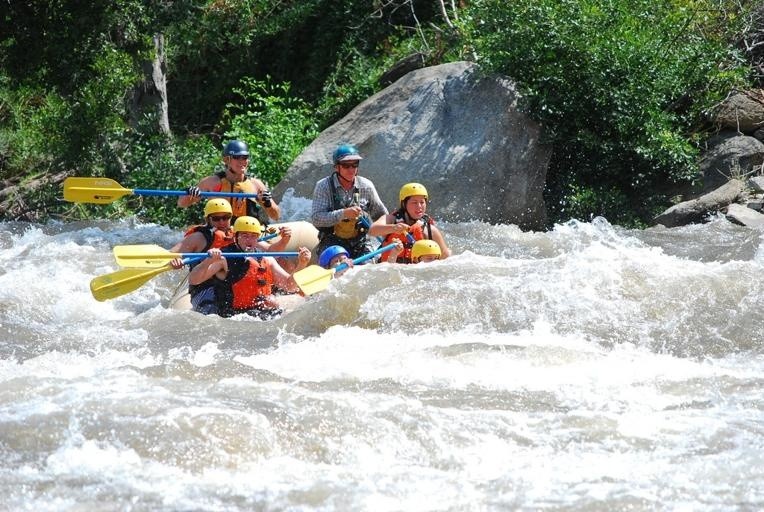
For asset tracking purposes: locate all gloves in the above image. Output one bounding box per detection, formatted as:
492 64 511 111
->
187 185 202 198
258 190 273 207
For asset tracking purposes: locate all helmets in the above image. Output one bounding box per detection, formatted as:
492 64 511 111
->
400 182 429 203
319 244 350 268
233 215 261 237
205 197 233 219
221 140 250 156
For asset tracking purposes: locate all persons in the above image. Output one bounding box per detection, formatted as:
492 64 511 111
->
168 197 292 315
317 238 405 278
187 215 312 322
178 139 281 225
177 241 181 245
368 182 450 264
311 144 390 264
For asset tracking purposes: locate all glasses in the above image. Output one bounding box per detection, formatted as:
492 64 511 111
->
209 213 230 222
339 162 359 168
232 154 249 160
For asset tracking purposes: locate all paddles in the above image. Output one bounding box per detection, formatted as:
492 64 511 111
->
292 242 397 297
62 176 258 204
112 244 300 269
89 231 281 302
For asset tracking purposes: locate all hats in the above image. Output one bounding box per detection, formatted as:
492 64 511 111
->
333 144 363 163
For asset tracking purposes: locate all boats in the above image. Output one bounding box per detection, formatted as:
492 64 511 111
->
168 221 321 310
